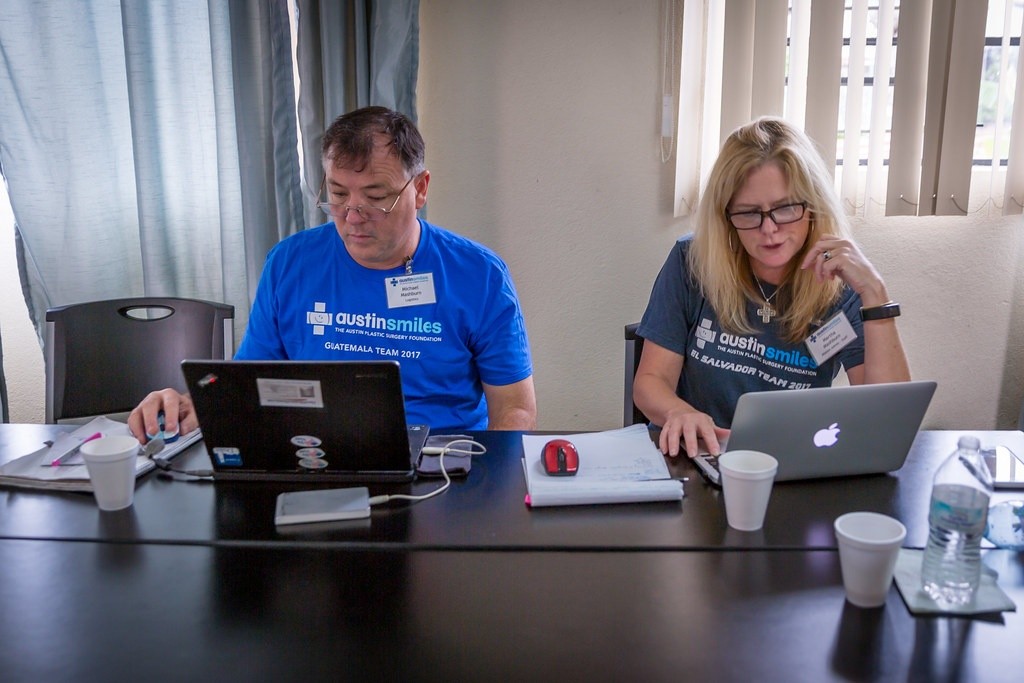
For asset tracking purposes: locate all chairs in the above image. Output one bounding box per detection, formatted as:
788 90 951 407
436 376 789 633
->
624 322 651 427
43 296 235 425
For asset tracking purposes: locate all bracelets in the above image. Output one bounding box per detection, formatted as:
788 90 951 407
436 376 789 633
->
859 300 901 322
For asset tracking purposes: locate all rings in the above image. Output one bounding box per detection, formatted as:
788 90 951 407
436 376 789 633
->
823 249 832 261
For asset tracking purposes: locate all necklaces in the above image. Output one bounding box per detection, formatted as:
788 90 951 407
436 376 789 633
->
751 266 789 324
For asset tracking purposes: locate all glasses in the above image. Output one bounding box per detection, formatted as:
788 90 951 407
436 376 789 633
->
725 201 808 230
315 173 414 221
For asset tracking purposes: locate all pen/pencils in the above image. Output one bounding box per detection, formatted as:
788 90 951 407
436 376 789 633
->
48 432 102 466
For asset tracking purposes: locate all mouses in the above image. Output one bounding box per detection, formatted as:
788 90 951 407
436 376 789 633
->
146 409 180 444
540 439 579 476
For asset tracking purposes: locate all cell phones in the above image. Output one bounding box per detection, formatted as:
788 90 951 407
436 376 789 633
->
959 445 1024 489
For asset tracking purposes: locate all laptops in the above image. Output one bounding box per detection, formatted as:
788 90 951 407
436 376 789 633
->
681 380 938 487
180 359 428 483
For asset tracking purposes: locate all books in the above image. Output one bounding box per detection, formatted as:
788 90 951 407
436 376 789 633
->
521 423 684 506
0 415 203 491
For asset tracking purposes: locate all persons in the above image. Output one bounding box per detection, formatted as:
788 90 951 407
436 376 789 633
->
633 118 912 457
129 106 537 443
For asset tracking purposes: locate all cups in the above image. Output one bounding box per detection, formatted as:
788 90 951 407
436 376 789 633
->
718 450 778 531
834 512 907 608
80 436 140 511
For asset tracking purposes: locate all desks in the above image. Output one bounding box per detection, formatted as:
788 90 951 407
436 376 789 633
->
0 423 1024 683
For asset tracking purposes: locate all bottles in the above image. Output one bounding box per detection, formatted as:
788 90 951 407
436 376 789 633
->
922 435 994 605
982 500 1024 551
